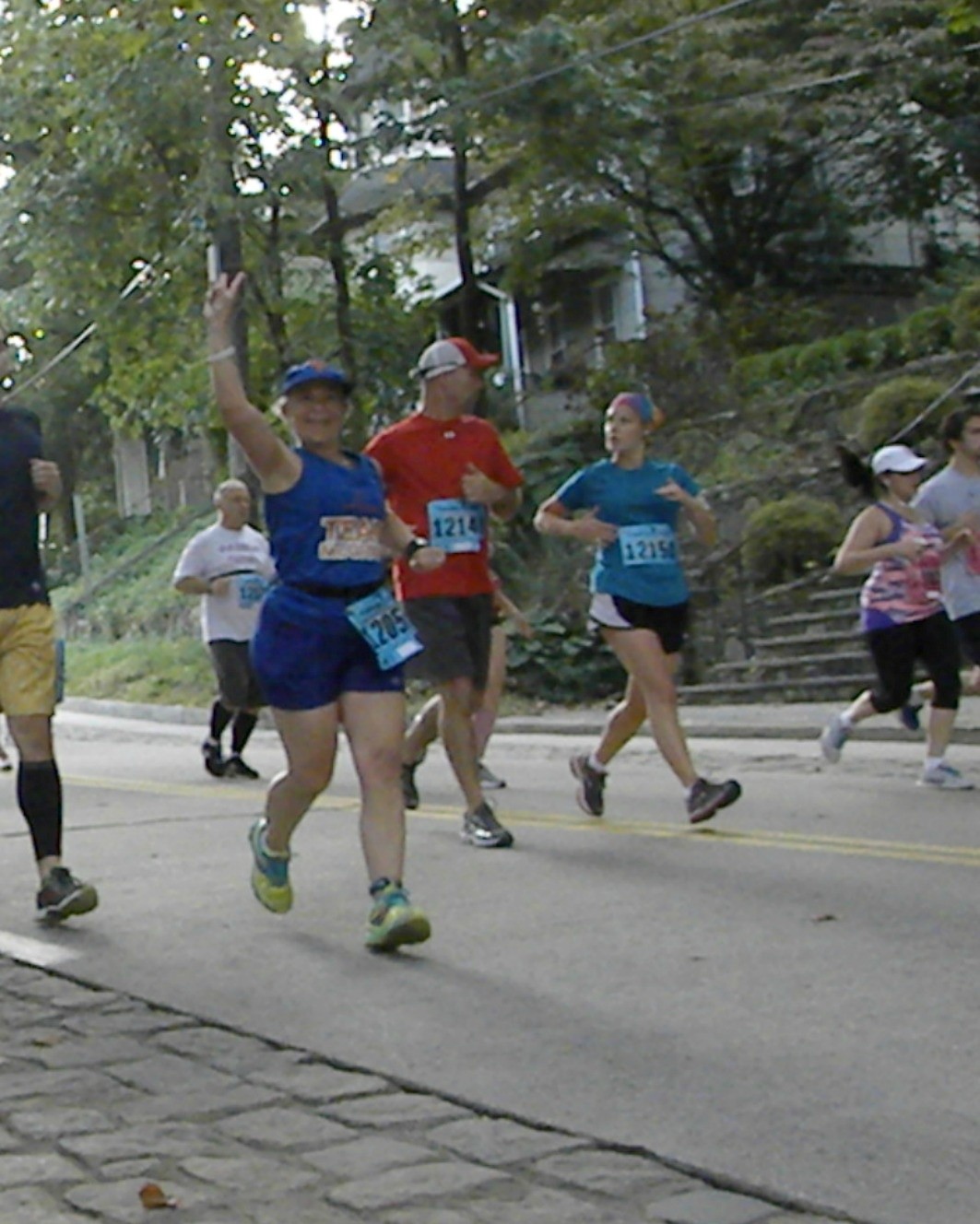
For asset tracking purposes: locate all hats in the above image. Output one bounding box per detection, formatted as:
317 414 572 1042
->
408 336 502 380
871 443 930 477
282 359 351 394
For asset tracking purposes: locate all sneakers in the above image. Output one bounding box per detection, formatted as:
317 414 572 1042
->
569 750 608 816
247 816 295 914
683 778 742 824
917 761 973 791
201 741 225 776
363 878 431 949
402 763 419 809
459 803 514 847
33 865 99 923
815 715 852 765
224 757 259 780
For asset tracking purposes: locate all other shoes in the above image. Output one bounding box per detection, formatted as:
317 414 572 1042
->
1 749 14 773
476 763 506 790
414 747 427 766
902 703 924 731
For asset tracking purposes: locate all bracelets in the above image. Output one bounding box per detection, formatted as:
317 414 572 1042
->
208 344 236 363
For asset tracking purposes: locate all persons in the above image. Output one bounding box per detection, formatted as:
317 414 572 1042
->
170 479 282 779
359 337 527 847
204 271 431 951
533 391 741 823
819 407 980 790
0 406 100 923
